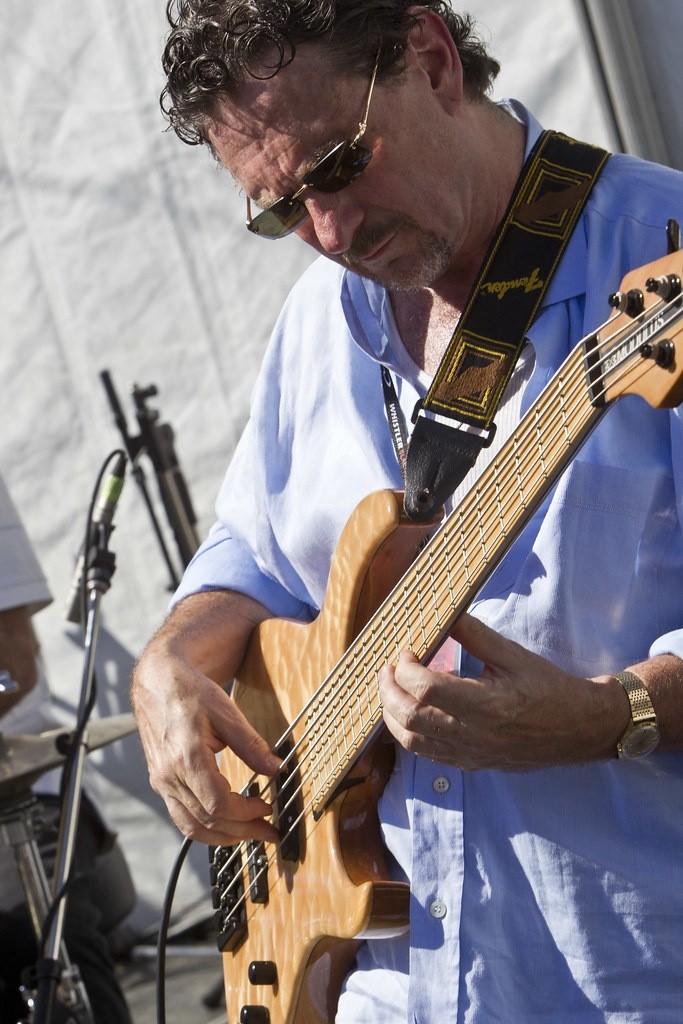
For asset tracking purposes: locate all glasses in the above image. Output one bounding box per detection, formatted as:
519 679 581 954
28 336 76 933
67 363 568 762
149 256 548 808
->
245 34 382 240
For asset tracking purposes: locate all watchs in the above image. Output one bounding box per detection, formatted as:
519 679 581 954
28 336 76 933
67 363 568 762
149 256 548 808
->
610 671 661 762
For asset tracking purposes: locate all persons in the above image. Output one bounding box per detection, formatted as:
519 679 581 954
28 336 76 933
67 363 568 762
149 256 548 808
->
1 478 98 1024
128 0 683 1024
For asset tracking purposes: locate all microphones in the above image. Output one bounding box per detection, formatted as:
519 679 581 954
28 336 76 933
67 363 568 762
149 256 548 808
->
65 454 131 624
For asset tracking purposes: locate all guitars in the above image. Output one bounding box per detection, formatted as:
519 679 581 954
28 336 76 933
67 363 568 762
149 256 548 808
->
208 221 683 1024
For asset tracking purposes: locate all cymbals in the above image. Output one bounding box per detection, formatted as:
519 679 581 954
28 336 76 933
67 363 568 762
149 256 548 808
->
0 709 140 809
